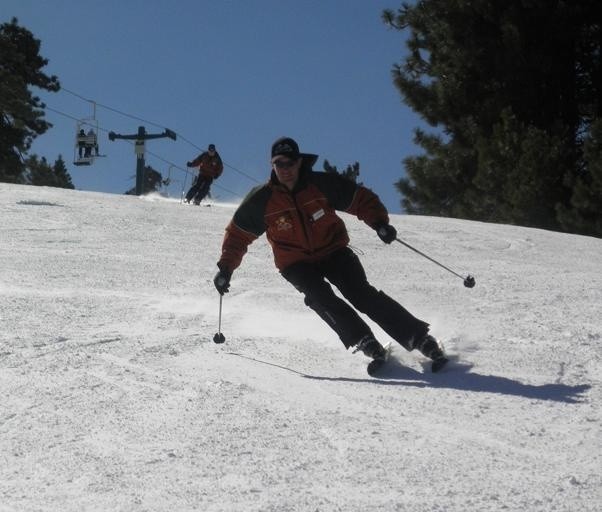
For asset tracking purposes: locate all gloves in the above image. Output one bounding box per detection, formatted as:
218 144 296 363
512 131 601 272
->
214 271 232 296
187 162 192 167
372 220 397 244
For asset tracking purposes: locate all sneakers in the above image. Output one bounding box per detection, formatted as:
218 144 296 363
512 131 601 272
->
421 337 451 373
359 336 388 376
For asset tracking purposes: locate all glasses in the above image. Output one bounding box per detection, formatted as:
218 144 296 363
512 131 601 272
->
275 160 295 168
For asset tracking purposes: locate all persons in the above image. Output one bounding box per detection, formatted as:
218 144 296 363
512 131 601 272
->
184 145 223 205
214 139 443 363
78 129 97 155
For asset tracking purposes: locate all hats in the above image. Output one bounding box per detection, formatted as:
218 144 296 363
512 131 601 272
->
272 136 300 161
209 144 216 152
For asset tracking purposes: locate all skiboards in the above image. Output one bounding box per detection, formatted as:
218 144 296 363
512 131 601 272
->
367 339 449 376
